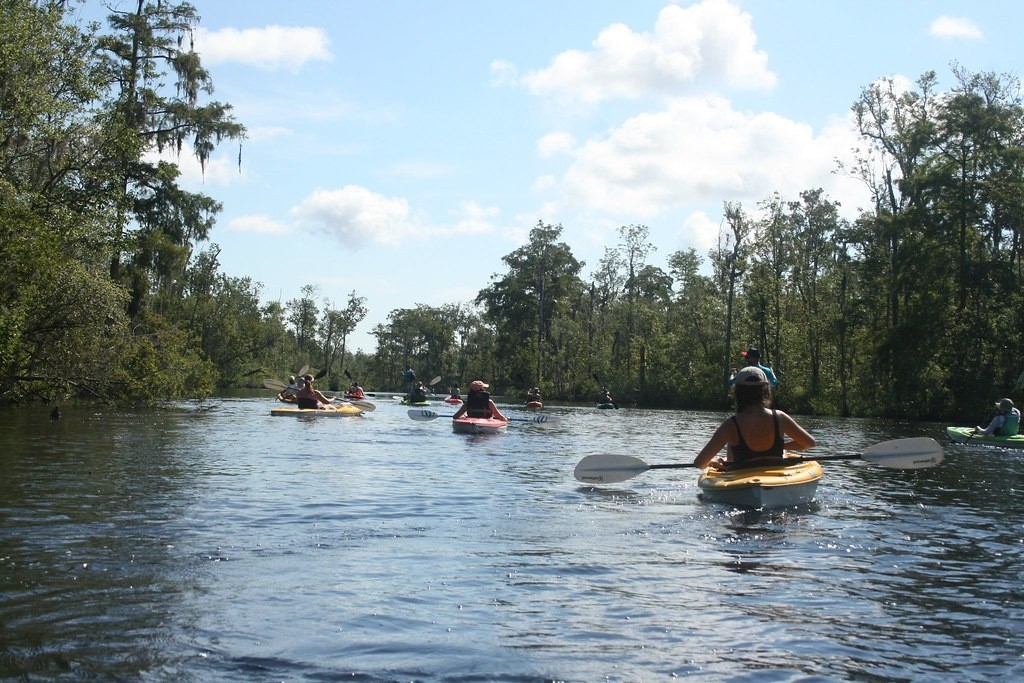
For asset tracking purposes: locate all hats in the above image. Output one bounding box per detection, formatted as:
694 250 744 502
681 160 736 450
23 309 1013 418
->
735 366 768 386
742 348 760 358
289 376 295 380
471 381 489 392
353 382 359 386
415 381 423 389
996 398 1014 411
303 375 314 382
532 388 540 393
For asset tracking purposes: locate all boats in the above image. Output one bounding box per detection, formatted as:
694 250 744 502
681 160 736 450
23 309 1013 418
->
271 402 363 416
445 395 463 404
947 426 1024 449
451 415 508 434
343 393 366 400
698 449 823 510
526 401 543 410
400 400 429 406
597 403 614 410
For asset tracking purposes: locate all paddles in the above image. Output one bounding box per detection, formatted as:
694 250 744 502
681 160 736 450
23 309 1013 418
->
593 373 619 409
344 369 352 380
399 375 442 405
314 370 328 379
963 406 998 445
365 393 375 397
573 432 947 486
407 409 561 429
275 364 310 401
263 378 377 412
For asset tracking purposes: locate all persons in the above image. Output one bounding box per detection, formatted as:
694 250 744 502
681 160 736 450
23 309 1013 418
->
453 381 508 421
728 348 778 389
599 387 612 402
693 367 817 469
297 374 337 409
401 365 461 401
280 375 365 399
976 398 1021 437
526 387 543 402
